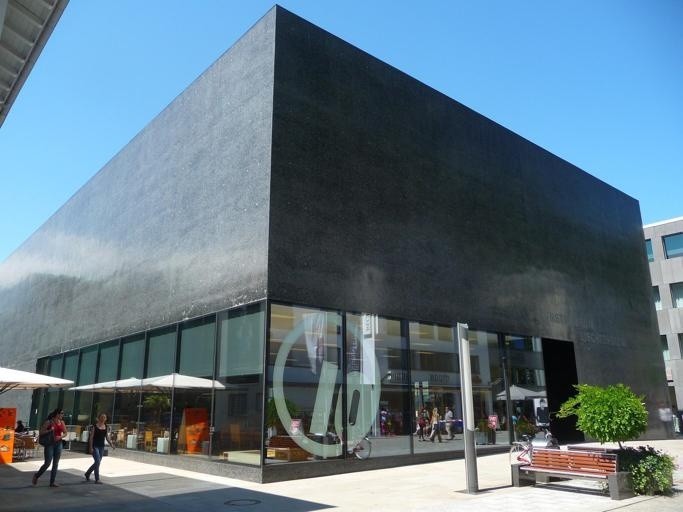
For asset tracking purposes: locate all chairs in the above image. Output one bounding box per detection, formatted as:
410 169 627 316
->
60 431 89 453
13 433 39 460
228 423 247 450
143 429 157 452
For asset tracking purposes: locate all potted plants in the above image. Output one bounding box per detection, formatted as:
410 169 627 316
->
514 417 538 441
629 454 676 496
474 419 496 445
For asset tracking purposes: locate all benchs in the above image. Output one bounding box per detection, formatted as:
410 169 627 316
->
510 444 635 499
265 434 308 461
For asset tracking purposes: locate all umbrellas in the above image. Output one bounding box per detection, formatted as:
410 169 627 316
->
493 384 546 401
139 372 226 392
0 367 75 394
67 376 158 393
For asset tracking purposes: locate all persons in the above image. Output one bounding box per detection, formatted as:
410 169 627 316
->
15 420 24 431
32 407 68 487
84 411 116 485
658 403 676 441
676 411 683 434
518 413 526 432
536 399 550 426
512 415 517 432
380 407 455 444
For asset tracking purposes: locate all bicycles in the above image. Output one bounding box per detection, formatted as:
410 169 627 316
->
509 426 561 465
349 422 372 461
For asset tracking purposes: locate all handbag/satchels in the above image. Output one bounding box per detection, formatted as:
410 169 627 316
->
38 420 55 447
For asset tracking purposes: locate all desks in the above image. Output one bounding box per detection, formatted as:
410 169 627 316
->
156 437 169 454
126 434 137 449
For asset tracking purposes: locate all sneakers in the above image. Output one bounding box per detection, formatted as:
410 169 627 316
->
94 479 102 485
84 472 91 481
31 473 39 487
49 482 59 487
